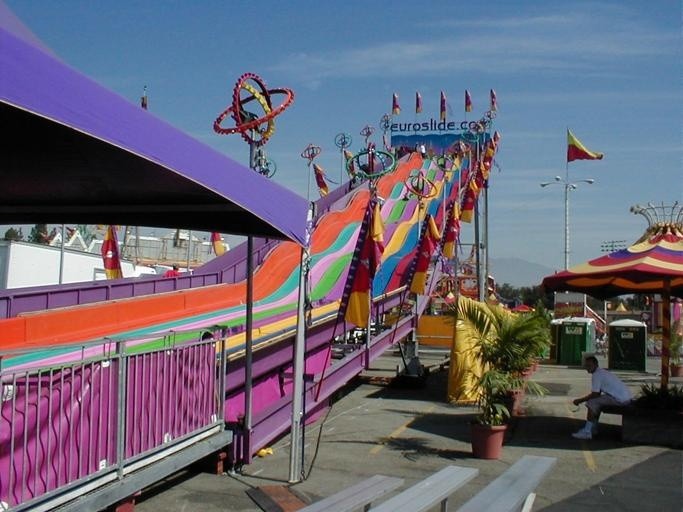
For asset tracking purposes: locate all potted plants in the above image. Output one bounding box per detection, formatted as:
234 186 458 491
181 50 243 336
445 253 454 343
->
669 318 683 377
429 285 555 460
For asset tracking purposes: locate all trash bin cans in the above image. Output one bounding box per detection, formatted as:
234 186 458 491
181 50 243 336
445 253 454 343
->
609 318 647 372
551 319 561 360
560 317 596 366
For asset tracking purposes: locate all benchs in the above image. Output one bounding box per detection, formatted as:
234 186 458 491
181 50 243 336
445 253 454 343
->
295 455 557 512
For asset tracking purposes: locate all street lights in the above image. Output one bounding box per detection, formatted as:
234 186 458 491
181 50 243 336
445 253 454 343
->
541 175 595 271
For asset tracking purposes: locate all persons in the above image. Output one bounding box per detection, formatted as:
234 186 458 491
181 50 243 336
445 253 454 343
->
568 355 632 439
162 263 181 277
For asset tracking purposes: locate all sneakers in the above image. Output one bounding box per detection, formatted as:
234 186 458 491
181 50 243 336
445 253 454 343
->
572 430 591 440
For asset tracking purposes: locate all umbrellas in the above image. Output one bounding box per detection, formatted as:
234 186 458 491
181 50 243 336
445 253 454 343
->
539 220 682 391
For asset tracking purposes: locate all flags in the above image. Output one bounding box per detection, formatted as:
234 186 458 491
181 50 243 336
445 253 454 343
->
381 133 392 154
344 150 356 183
490 90 498 114
567 128 603 165
310 163 328 198
440 90 445 122
335 198 386 330
401 212 441 298
391 94 400 116
458 130 499 225
464 89 472 112
437 198 461 260
99 225 124 280
211 232 225 257
414 90 422 114
